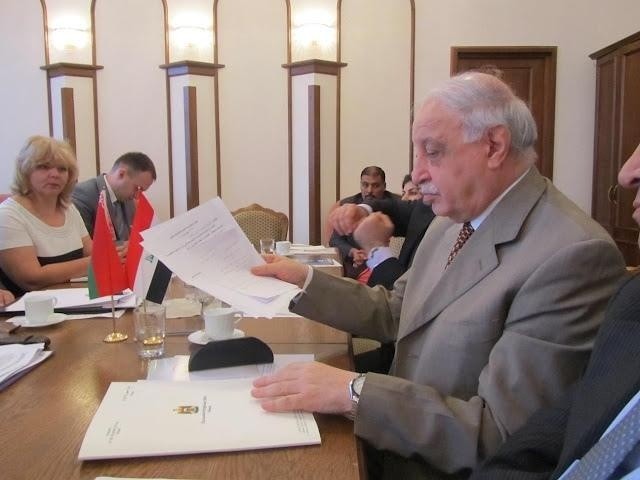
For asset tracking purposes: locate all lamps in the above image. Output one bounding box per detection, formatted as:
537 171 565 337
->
283 0 342 64
38 0 97 68
162 1 220 66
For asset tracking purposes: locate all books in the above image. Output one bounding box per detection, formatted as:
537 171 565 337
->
78 381 321 460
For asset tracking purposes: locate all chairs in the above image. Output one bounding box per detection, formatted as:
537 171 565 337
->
229 202 289 253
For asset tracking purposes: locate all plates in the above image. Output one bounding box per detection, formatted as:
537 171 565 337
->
14 312 68 329
273 252 296 258
188 326 246 347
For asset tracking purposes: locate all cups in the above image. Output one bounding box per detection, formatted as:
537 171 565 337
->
203 308 247 342
259 238 274 257
277 241 295 255
196 289 223 320
21 292 57 325
132 303 169 360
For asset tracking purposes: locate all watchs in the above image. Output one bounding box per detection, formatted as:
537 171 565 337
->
346 372 367 422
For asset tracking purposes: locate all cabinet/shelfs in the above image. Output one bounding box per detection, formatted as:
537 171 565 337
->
586 31 639 272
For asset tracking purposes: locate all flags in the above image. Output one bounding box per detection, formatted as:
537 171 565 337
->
87 191 129 299
124 192 174 304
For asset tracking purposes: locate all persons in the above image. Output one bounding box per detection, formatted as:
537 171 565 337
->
0 136 129 292
0 281 15 309
467 144 640 480
74 152 156 240
324 165 436 374
250 68 627 480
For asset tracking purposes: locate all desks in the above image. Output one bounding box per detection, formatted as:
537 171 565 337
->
0 241 355 346
0 337 368 480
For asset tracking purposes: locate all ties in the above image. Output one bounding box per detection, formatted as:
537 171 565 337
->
562 396 637 473
448 222 472 267
